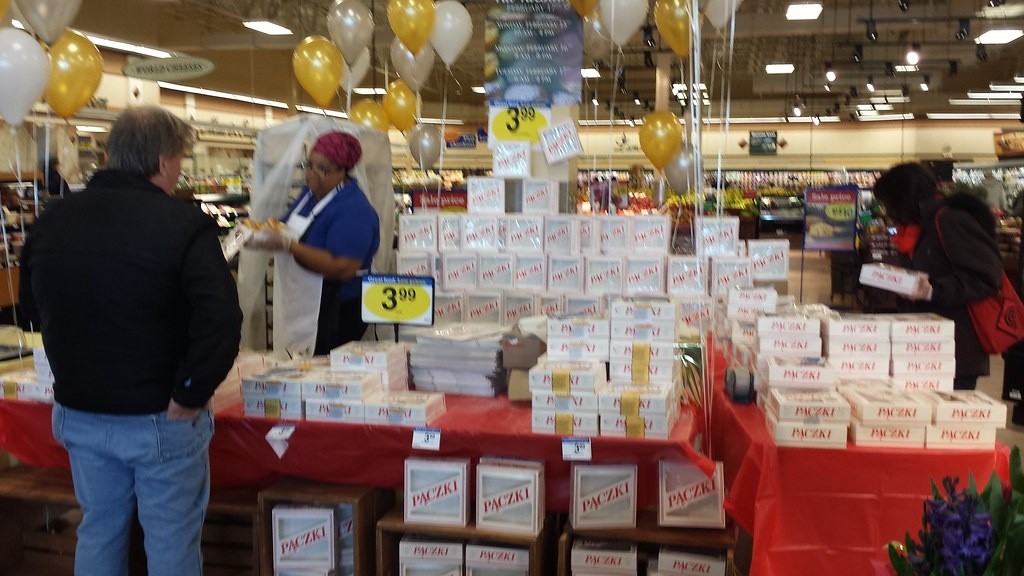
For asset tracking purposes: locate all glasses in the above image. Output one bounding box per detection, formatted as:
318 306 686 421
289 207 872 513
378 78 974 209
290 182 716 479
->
301 160 339 175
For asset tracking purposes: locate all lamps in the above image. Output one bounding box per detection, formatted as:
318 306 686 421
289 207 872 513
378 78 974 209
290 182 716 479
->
852 44 865 63
906 42 922 65
640 24 659 47
11 18 172 59
898 0 911 12
765 64 796 74
866 75 875 93
955 18 970 40
785 3 823 21
591 91 600 107
919 72 930 92
867 19 879 41
811 113 821 126
976 43 990 63
824 62 837 82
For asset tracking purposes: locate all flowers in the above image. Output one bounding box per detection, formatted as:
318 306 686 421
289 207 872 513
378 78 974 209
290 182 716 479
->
888 445 1024 576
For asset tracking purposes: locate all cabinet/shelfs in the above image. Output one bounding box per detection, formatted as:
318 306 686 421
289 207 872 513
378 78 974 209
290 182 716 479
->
375 509 541 576
0 465 258 576
0 107 1024 271
256 484 395 576
557 515 739 576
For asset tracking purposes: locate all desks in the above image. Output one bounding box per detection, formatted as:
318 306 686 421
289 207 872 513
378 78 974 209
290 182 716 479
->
0 381 717 491
702 331 1011 576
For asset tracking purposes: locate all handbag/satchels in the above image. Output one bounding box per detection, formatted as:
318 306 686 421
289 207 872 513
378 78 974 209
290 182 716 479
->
936 206 1024 354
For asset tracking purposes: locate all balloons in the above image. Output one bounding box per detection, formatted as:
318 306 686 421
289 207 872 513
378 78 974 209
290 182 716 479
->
350 97 388 133
704 0 742 29
331 41 371 94
389 36 435 92
664 141 704 196
386 0 435 55
654 0 694 59
292 35 343 108
571 0 649 61
0 0 103 127
428 0 472 66
409 124 441 170
382 80 423 132
327 0 374 66
639 110 682 175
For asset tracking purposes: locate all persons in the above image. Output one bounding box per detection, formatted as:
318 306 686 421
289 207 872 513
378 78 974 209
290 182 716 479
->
235 130 381 358
17 106 244 576
42 156 72 200
871 161 1002 389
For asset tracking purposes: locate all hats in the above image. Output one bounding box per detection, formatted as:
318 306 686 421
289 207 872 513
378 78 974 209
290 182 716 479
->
313 131 362 171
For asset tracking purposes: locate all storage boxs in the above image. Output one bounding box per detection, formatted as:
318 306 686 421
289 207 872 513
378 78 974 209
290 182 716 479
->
221 178 1006 451
